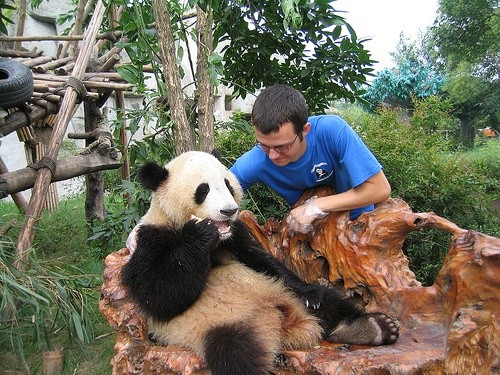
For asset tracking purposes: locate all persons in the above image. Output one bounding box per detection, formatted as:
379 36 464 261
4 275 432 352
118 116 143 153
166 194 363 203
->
126 84 392 256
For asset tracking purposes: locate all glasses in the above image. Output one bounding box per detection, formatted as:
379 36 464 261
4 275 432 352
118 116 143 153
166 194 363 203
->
254 128 304 154
